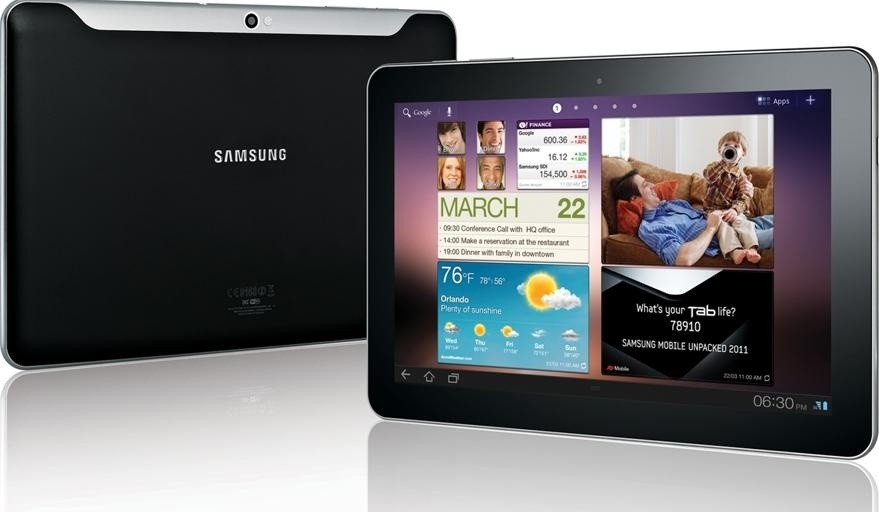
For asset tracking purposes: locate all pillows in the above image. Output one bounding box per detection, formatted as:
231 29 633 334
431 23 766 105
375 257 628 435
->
602 154 773 234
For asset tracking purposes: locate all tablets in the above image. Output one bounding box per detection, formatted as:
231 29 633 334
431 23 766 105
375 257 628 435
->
365 45 879 459
0 0 456 371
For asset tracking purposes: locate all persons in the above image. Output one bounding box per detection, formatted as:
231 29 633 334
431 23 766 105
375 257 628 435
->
610 168 774 267
477 121 505 155
438 158 466 190
477 157 505 191
702 132 762 264
437 122 466 155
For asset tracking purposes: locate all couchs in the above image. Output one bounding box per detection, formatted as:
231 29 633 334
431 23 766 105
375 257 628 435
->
601 156 774 270
